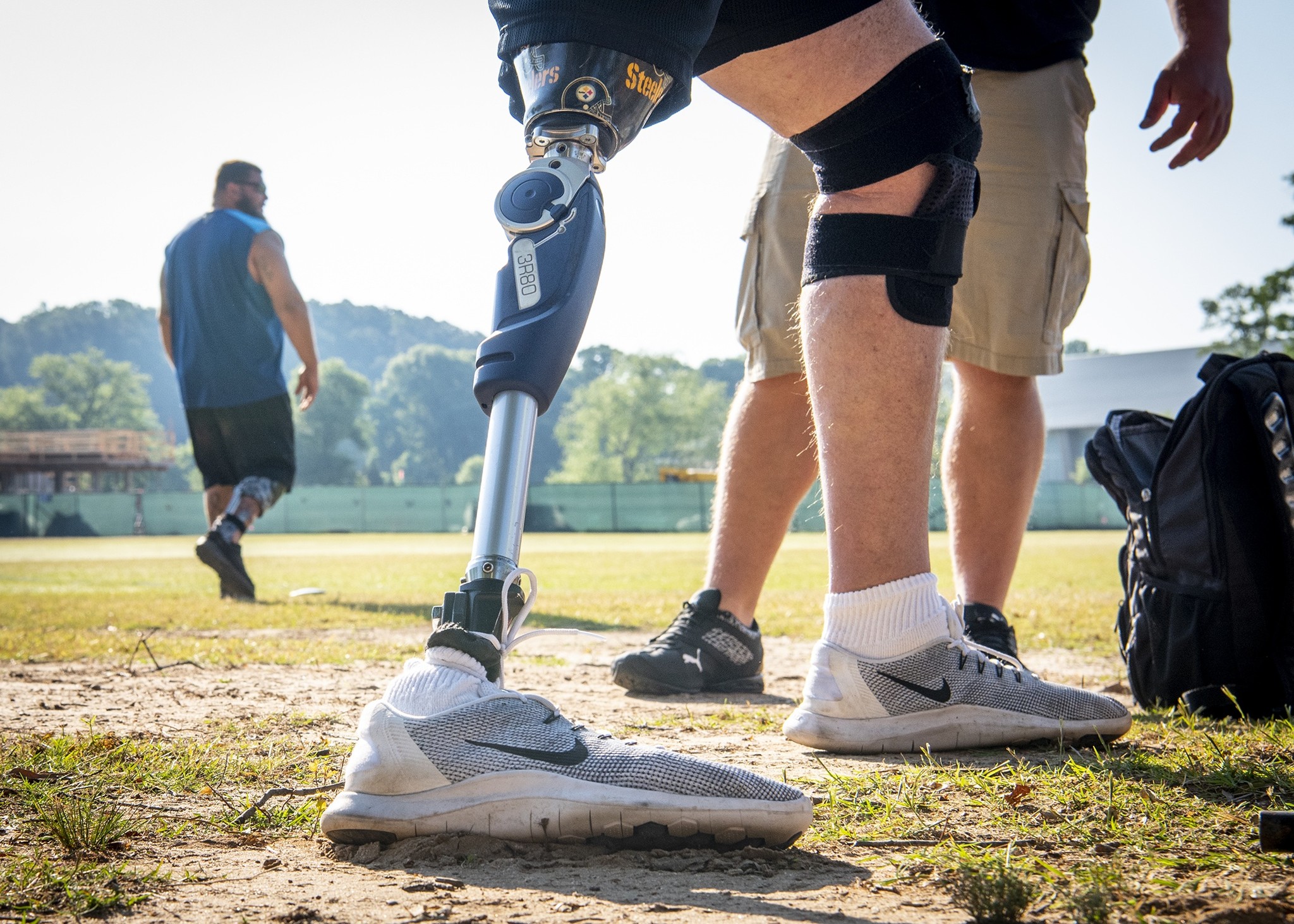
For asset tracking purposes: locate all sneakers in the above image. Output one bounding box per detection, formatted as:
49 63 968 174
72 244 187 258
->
781 595 1132 752
611 586 766 694
962 603 1018 664
321 567 813 857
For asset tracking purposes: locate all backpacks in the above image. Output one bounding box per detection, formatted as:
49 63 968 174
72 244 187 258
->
1085 346 1294 729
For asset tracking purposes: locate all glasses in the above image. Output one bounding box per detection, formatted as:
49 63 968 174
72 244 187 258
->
234 180 266 195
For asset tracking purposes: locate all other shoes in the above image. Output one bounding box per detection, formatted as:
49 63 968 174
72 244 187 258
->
194 529 256 600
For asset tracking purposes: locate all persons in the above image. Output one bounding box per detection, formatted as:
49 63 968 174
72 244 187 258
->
320 0 1132 847
159 159 323 603
607 1 1235 701
